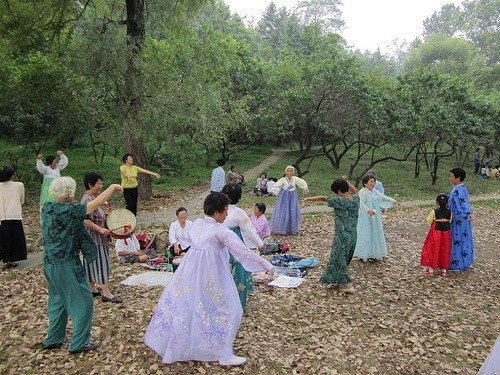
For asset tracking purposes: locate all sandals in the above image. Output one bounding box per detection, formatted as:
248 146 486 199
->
422 270 435 277
440 270 448 277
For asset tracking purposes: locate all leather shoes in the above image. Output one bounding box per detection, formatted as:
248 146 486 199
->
69 344 98 354
6 262 19 268
92 289 103 297
43 343 64 349
101 295 125 303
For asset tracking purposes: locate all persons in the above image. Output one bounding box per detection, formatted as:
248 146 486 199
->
222 184 270 317
270 165 309 236
210 159 227 192
40 176 124 353
81 171 123 303
447 167 475 271
227 165 246 186
475 148 482 173
115 234 157 264
254 173 279 197
168 207 192 273
144 192 276 366
0 166 27 269
36 150 68 225
481 161 500 180
120 153 161 216
420 193 452 277
365 171 385 213
300 175 361 293
354 175 400 263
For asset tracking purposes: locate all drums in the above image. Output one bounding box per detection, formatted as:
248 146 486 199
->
105 208 136 238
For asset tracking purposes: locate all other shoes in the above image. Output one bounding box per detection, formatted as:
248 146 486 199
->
368 257 377 263
219 355 248 366
339 287 357 294
